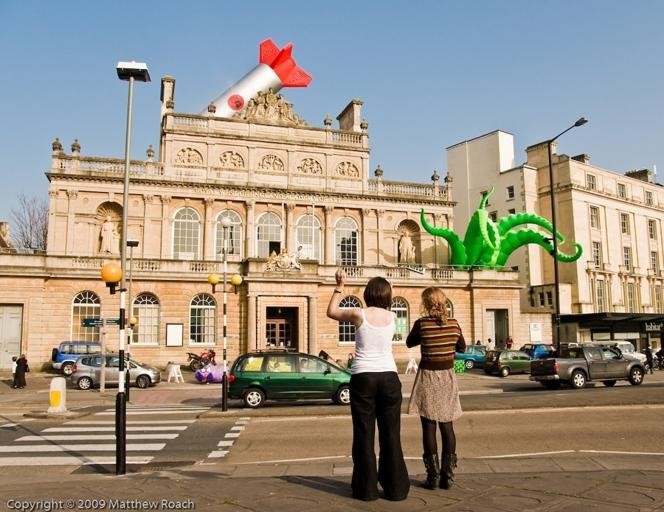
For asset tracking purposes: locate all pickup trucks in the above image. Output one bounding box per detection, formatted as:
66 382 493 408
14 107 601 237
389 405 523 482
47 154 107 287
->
528 344 645 394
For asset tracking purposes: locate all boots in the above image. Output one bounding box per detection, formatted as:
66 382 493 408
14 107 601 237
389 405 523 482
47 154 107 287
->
439 454 457 489
421 454 440 490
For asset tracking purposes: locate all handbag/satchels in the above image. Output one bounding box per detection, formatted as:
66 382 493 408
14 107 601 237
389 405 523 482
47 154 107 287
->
25 365 29 372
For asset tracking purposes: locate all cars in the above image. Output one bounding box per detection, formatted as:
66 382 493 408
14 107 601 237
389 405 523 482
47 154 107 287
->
452 343 488 371
519 341 552 360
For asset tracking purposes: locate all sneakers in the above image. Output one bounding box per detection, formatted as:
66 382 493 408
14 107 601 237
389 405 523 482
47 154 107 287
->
13 385 24 388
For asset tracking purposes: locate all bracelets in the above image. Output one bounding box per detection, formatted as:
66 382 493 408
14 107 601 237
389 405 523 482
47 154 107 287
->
333 289 341 293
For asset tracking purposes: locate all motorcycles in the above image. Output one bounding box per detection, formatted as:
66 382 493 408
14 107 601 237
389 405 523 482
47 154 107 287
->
185 348 216 371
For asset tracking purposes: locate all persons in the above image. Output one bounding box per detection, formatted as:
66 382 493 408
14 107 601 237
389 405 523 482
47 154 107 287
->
405 285 467 490
286 338 297 353
505 336 514 349
231 87 307 127
398 230 416 264
99 215 117 253
11 356 18 386
273 362 281 372
12 353 27 388
475 340 481 345
612 343 621 355
346 352 353 372
485 338 494 350
299 358 308 372
174 147 359 178
644 345 654 374
325 267 411 501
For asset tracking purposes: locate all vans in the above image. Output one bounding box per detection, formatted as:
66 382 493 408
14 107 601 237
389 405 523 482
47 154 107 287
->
583 339 651 374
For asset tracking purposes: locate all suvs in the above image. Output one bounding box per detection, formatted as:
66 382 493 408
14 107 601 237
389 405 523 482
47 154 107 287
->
69 351 160 391
482 347 535 377
227 350 353 405
47 340 110 376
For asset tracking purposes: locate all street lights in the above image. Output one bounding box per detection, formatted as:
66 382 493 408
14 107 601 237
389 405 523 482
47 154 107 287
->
124 235 141 402
541 116 589 354
207 212 243 411
100 58 151 475
